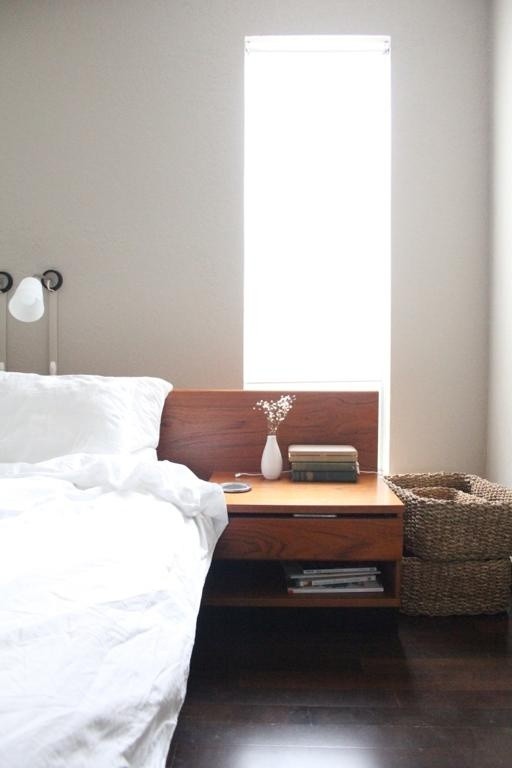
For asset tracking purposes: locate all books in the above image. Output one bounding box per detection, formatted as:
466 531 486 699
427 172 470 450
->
284 563 385 595
288 443 360 483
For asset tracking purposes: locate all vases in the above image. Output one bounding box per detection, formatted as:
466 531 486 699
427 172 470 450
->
260 435 283 480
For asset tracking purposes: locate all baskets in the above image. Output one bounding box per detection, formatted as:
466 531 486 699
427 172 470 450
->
381 471 512 562
400 557 511 617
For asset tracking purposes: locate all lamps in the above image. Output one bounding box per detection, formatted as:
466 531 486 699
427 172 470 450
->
8 273 48 323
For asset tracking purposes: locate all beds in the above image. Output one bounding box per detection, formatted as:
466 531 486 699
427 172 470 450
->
0 455 230 768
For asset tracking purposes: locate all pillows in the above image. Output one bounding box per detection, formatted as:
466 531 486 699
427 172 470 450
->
0 371 173 462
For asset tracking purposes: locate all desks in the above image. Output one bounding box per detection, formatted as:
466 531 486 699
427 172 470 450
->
200 470 405 634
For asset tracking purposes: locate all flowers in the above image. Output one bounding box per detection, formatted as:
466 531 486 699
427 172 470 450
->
253 395 297 436
